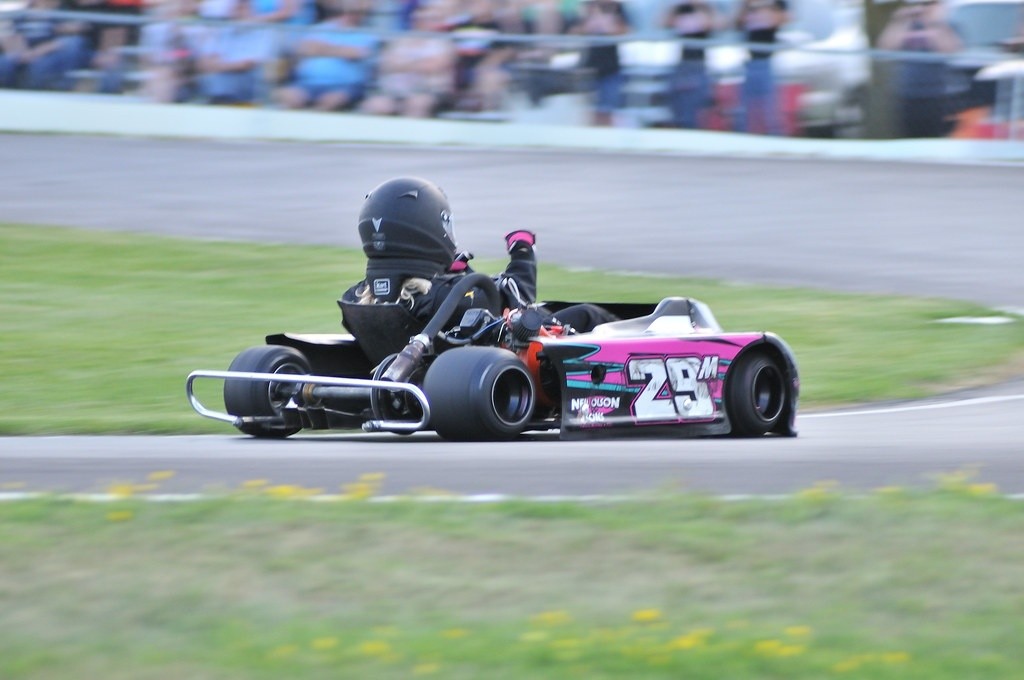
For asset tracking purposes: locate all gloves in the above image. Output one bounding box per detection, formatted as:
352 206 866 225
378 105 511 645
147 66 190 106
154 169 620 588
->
448 253 473 274
506 230 536 255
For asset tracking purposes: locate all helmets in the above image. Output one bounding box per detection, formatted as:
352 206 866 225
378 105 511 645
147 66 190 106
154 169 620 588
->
358 175 457 264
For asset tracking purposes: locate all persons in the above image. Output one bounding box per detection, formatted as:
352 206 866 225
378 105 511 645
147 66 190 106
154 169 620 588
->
877 0 968 137
0 0 789 134
341 176 622 333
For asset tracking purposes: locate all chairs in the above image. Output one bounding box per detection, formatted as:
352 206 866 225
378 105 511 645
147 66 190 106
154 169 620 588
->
337 299 472 367
